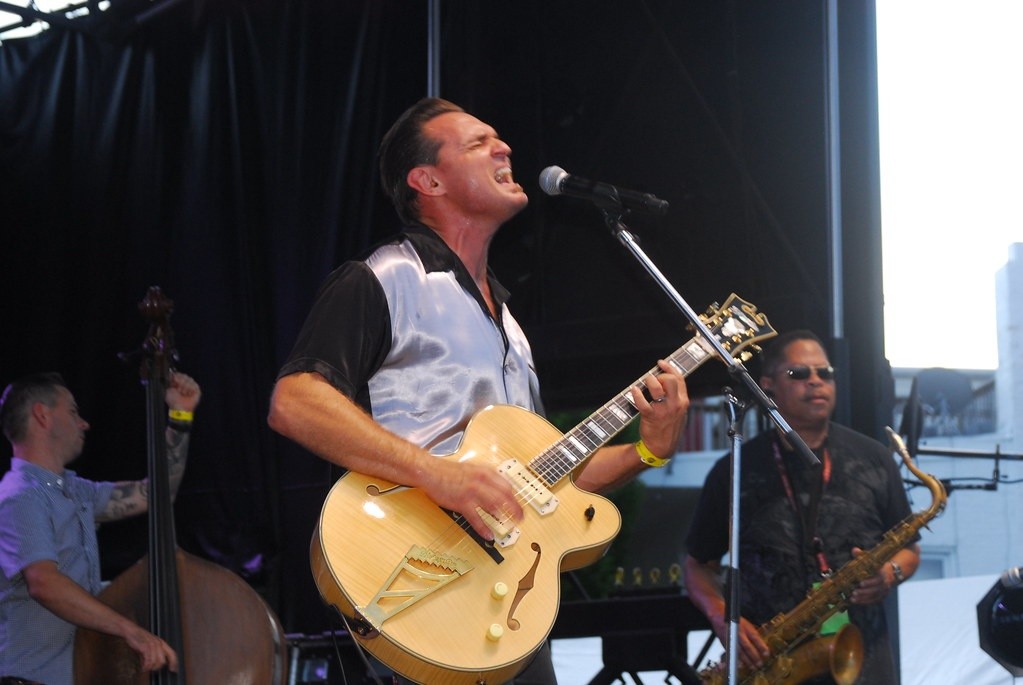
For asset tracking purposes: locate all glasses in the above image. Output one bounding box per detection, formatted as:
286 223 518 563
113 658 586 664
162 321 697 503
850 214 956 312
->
769 367 834 380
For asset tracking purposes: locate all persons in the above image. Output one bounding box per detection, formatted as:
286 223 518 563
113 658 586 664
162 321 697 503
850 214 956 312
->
0 370 201 685
266 93 691 685
684 330 922 685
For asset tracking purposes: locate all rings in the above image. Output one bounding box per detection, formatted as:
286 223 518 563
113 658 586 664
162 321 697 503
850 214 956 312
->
653 396 666 403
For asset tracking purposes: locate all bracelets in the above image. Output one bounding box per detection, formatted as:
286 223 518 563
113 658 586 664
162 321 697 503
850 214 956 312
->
167 409 194 432
635 439 672 468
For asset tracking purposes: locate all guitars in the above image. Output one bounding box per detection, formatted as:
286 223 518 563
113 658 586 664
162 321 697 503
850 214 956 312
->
302 290 782 685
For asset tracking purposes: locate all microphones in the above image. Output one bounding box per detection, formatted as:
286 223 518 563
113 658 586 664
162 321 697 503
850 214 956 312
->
539 165 670 216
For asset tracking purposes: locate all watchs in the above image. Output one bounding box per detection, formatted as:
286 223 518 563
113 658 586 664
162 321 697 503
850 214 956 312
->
889 559 905 584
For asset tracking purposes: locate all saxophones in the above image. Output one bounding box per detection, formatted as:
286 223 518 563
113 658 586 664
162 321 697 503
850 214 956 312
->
697 424 954 684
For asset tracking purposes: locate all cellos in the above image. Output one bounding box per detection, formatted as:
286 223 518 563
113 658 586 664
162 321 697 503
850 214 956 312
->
67 277 293 685
